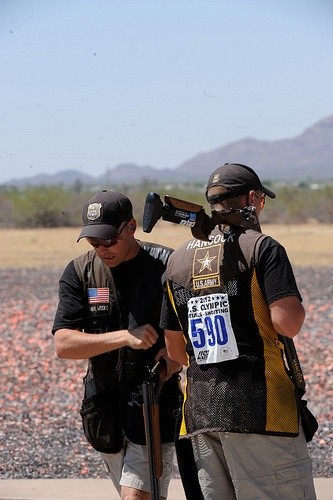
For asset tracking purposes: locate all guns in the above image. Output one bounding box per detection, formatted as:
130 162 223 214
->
143 192 262 233
128 356 167 500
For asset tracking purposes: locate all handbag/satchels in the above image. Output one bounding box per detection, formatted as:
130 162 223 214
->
79 392 123 454
301 400 319 443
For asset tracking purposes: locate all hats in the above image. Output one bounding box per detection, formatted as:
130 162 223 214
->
205 162 276 204
77 189 133 243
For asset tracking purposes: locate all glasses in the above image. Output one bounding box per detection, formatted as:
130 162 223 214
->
86 217 133 248
255 193 265 209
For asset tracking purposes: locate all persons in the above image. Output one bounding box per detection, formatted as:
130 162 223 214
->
51 190 185 499
175 357 207 500
160 161 318 500
